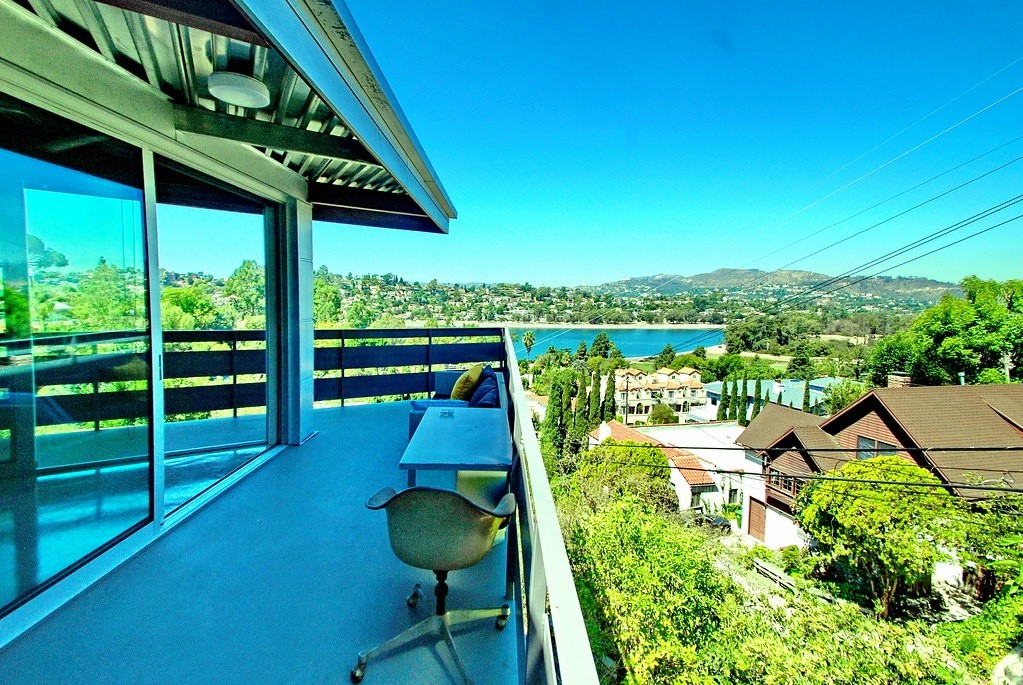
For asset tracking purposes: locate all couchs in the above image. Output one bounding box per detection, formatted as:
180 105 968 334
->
407 368 508 446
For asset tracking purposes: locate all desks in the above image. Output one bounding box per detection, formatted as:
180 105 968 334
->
399 405 514 501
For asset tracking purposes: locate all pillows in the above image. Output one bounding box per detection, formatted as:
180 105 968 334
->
409 399 470 409
476 364 495 388
450 362 483 399
471 377 497 404
474 387 499 408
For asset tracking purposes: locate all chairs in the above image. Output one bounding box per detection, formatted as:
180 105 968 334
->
349 484 517 685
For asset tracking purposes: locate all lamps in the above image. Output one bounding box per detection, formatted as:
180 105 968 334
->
207 71 270 108
